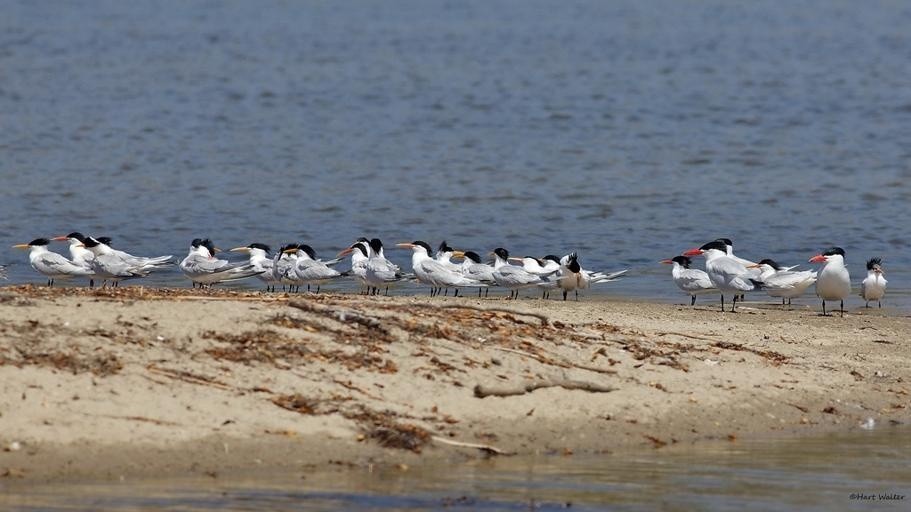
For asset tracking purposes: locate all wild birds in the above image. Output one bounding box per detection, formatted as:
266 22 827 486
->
808 247 852 318
179 237 629 301
12 237 96 289
49 231 176 290
858 257 888 308
657 238 818 313
